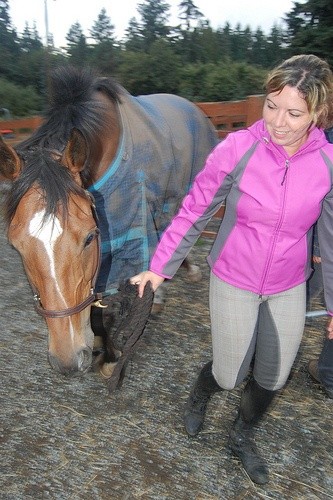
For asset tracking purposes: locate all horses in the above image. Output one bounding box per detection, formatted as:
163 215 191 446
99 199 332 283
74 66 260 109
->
0 61 220 383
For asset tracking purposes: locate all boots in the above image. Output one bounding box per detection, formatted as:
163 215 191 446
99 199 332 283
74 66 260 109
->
228 372 288 485
184 360 225 436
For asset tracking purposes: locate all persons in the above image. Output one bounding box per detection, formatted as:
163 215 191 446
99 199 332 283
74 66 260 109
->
130 55 333 484
